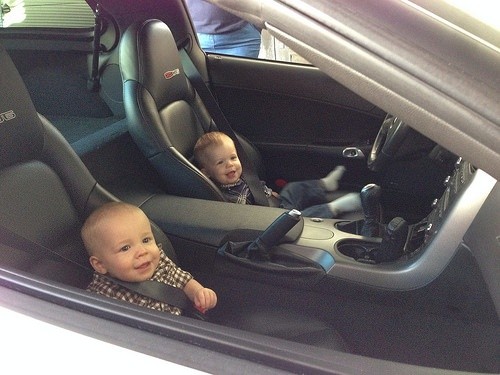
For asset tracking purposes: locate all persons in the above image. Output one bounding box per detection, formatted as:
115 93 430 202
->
183 0 263 61
194 130 364 220
79 201 218 326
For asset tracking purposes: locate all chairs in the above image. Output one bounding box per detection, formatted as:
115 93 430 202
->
0 51 348 361
120 19 363 219
